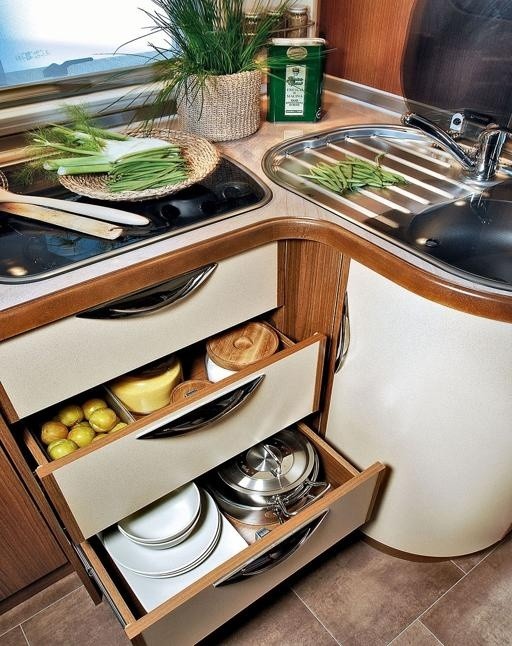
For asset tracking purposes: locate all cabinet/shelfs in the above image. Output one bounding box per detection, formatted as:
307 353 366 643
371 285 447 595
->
1 241 387 646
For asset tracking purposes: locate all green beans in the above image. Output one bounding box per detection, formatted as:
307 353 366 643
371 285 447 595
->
298 150 408 193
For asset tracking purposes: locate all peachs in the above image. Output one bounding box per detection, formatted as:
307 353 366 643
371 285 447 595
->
40 397 130 459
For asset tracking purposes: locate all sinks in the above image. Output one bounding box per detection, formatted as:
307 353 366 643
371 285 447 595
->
409 179 512 289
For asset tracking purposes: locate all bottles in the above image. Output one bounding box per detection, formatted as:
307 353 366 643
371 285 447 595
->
244 5 310 51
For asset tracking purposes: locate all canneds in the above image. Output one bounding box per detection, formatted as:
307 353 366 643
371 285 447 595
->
245 7 308 37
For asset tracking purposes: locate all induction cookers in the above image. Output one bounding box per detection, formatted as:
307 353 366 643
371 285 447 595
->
0 149 274 285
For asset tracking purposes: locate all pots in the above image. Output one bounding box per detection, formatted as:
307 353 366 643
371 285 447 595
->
207 428 331 525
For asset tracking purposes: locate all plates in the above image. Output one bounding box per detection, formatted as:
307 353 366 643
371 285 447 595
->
97 509 249 614
99 491 224 579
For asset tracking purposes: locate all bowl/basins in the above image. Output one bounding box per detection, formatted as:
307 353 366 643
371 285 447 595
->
117 473 203 551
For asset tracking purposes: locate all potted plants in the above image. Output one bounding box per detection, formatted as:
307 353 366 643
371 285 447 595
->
96 0 339 142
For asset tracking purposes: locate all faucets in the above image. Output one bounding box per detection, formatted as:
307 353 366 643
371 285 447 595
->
401 109 508 185
